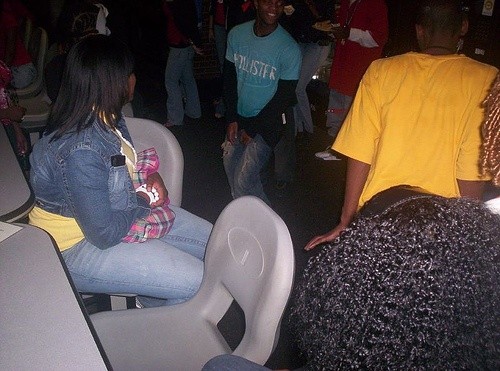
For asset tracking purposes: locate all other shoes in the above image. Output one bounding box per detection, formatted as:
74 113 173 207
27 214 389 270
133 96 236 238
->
314 145 343 162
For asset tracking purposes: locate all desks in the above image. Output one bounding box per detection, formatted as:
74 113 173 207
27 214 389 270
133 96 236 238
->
0 121 36 224
1 223 113 371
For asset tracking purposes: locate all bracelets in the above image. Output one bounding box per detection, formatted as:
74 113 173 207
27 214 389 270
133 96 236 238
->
209 27 213 31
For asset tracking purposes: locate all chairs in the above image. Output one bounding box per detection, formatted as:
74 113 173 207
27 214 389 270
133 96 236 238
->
9 16 51 138
106 116 183 309
92 195 295 371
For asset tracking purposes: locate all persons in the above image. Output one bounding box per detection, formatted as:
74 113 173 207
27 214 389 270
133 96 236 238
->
315 1 389 161
291 1 329 136
0 57 28 188
161 0 204 127
222 0 301 210
27 35 213 307
304 1 499 252
208 0 229 83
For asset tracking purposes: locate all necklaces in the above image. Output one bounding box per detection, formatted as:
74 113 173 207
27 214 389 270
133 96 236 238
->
344 0 358 26
418 44 455 54
